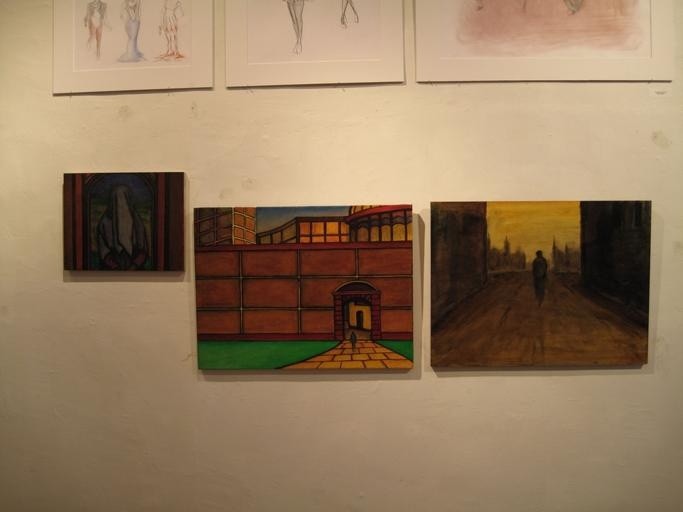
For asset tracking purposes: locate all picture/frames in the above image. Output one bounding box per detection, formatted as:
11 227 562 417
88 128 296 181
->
48 1 214 92
413 0 673 85
224 2 406 90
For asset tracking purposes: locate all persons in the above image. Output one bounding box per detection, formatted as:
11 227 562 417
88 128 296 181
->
530 250 549 306
157 0 184 65
287 1 311 54
348 332 360 357
80 0 114 61
119 0 148 65
339 1 360 30
